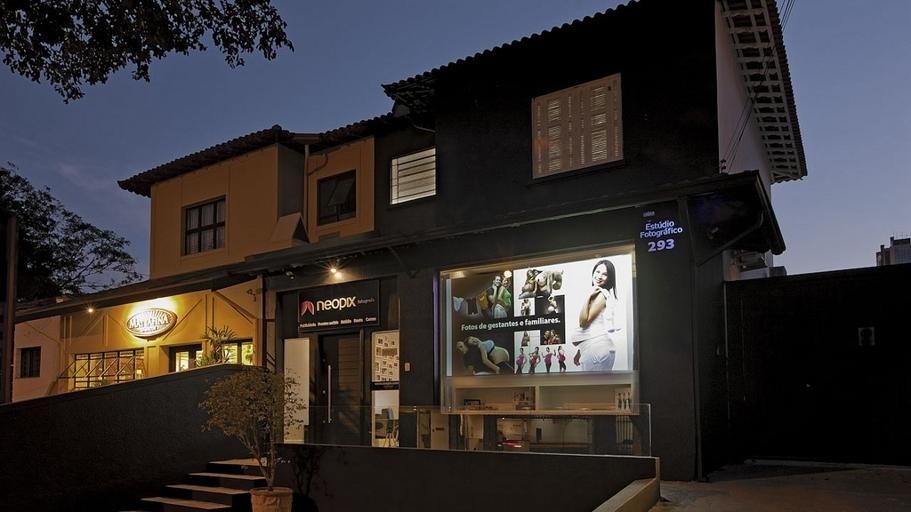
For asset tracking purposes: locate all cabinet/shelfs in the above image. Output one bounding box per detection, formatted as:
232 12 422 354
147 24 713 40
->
440 379 642 420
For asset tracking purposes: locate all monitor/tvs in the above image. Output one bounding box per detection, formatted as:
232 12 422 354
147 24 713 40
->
381 408 394 419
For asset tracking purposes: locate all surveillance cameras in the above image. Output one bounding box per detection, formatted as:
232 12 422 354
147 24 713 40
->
285 270 296 282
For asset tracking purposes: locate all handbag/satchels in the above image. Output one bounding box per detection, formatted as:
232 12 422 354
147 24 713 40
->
602 289 623 332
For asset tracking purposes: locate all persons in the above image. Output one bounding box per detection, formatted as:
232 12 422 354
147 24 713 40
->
572 258 619 373
492 275 512 321
522 330 531 347
454 341 494 375
515 347 527 375
519 267 564 317
465 335 514 374
481 273 502 322
516 394 528 405
548 330 562 344
542 329 558 345
557 345 567 372
542 346 557 373
528 347 541 373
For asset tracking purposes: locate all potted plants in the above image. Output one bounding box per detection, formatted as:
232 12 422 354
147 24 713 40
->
195 363 314 512
